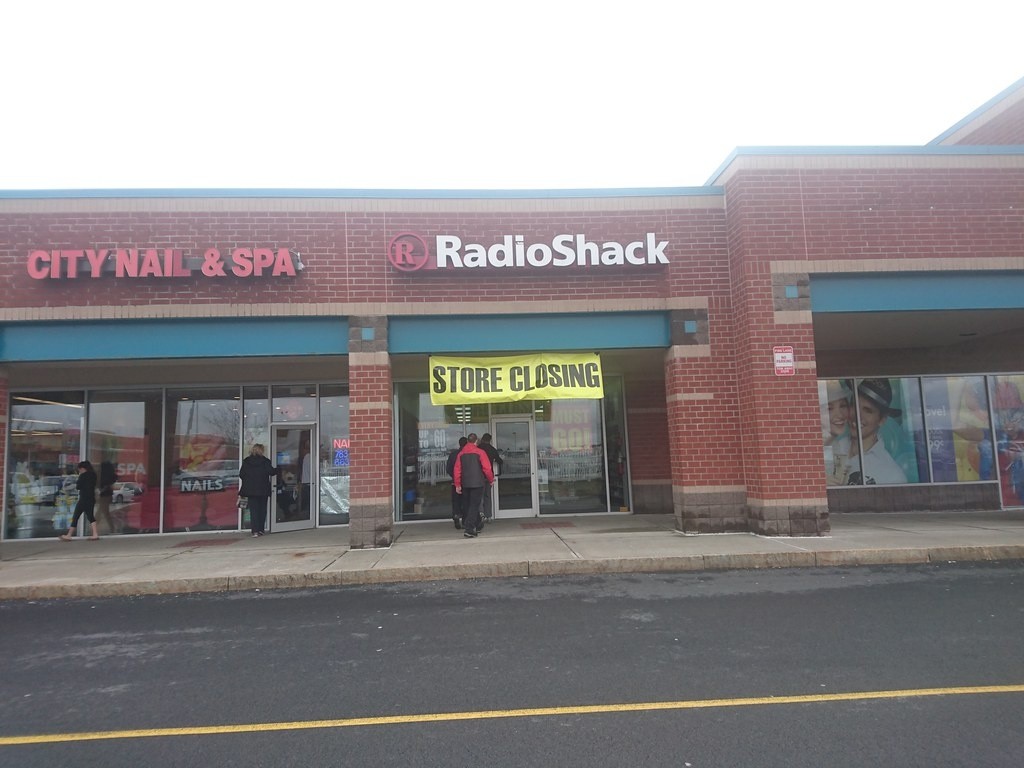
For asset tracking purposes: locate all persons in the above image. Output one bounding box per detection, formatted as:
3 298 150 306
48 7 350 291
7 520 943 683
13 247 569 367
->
59 460 97 541
453 433 494 538
827 378 907 485
301 446 311 510
240 444 280 537
447 437 467 529
477 433 503 521
95 461 116 533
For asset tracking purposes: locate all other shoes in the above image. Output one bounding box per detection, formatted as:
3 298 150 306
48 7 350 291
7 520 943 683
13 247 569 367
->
474 528 481 533
259 530 265 535
86 537 99 541
251 532 259 538
464 530 477 537
461 520 466 529
62 535 73 541
479 512 485 522
486 517 492 524
454 515 461 529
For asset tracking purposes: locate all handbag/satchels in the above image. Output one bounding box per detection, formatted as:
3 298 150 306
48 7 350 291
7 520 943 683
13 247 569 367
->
236 496 248 509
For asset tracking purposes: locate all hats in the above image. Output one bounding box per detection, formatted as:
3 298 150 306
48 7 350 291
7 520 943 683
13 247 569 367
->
845 378 902 418
995 382 1024 409
817 380 848 405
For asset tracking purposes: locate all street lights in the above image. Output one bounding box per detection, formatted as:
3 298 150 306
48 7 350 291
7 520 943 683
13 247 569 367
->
512 432 517 451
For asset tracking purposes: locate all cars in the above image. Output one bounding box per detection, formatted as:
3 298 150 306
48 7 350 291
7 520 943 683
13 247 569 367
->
62 474 79 501
178 460 240 486
17 475 67 502
9 472 33 500
96 482 141 504
